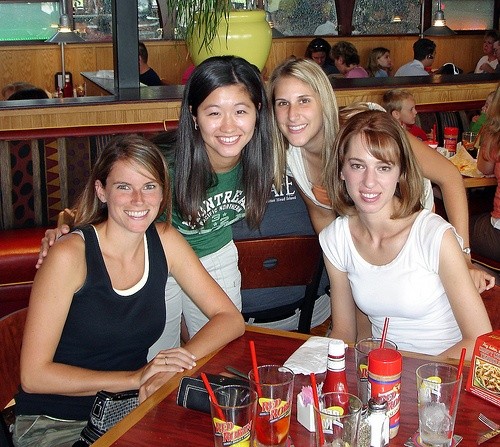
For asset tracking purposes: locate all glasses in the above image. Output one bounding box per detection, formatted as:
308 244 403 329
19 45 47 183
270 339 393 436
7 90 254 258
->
309 44 328 48
443 62 459 75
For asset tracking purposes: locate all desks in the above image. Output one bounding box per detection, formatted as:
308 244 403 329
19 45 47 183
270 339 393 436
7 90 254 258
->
462 176 496 187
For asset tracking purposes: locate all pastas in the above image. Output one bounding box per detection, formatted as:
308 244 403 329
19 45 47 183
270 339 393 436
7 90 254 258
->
477 363 500 393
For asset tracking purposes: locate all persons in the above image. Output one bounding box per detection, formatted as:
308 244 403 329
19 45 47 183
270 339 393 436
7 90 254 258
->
318 109 493 361
265 57 496 295
35 55 273 371
304 31 500 253
137 41 195 87
11 134 246 447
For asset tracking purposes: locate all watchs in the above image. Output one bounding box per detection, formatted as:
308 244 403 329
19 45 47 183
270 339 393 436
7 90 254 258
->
460 247 472 254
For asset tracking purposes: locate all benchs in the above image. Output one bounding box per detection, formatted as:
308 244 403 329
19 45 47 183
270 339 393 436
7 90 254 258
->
0 121 179 312
413 100 486 150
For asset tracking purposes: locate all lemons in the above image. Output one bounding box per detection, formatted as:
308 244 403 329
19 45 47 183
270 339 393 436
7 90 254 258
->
276 416 289 443
228 439 257 447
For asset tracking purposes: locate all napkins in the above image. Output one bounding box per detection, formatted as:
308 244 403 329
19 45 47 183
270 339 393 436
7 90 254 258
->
278 336 349 376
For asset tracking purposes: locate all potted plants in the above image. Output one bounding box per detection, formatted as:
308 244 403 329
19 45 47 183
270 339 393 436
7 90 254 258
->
164 0 273 85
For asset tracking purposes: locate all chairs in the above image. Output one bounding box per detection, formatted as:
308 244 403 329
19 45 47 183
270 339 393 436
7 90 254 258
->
0 307 29 411
234 234 323 334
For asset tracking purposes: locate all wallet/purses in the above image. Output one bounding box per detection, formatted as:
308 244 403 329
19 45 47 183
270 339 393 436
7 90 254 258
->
176 372 250 420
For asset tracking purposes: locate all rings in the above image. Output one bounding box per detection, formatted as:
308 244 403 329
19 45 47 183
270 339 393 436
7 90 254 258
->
164 356 168 365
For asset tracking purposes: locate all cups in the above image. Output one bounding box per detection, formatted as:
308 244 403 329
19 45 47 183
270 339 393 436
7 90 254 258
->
462 132 480 158
416 363 462 447
248 364 295 447
76 81 86 97
313 392 363 447
209 385 259 447
355 337 398 407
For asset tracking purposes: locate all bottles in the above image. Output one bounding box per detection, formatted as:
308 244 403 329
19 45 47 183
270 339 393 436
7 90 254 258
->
63 75 72 98
368 349 403 440
342 405 371 447
444 127 458 157
321 339 350 443
366 397 390 447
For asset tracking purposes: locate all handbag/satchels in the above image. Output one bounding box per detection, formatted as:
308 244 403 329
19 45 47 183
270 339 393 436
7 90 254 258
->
78 389 140 447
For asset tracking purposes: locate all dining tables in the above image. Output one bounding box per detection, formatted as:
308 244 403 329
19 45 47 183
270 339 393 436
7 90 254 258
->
89 325 500 447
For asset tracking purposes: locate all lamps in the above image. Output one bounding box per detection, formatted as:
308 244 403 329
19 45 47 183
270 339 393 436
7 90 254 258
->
43 0 86 43
418 1 457 37
